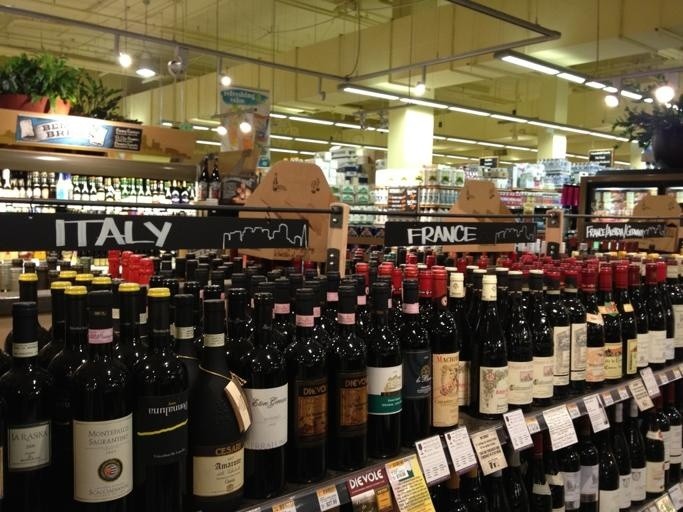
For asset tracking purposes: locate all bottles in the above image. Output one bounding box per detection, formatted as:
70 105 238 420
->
348 227 385 239
0 156 224 216
375 157 606 208
0 245 683 512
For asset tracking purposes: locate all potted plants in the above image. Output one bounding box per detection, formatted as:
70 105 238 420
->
0 52 83 115
